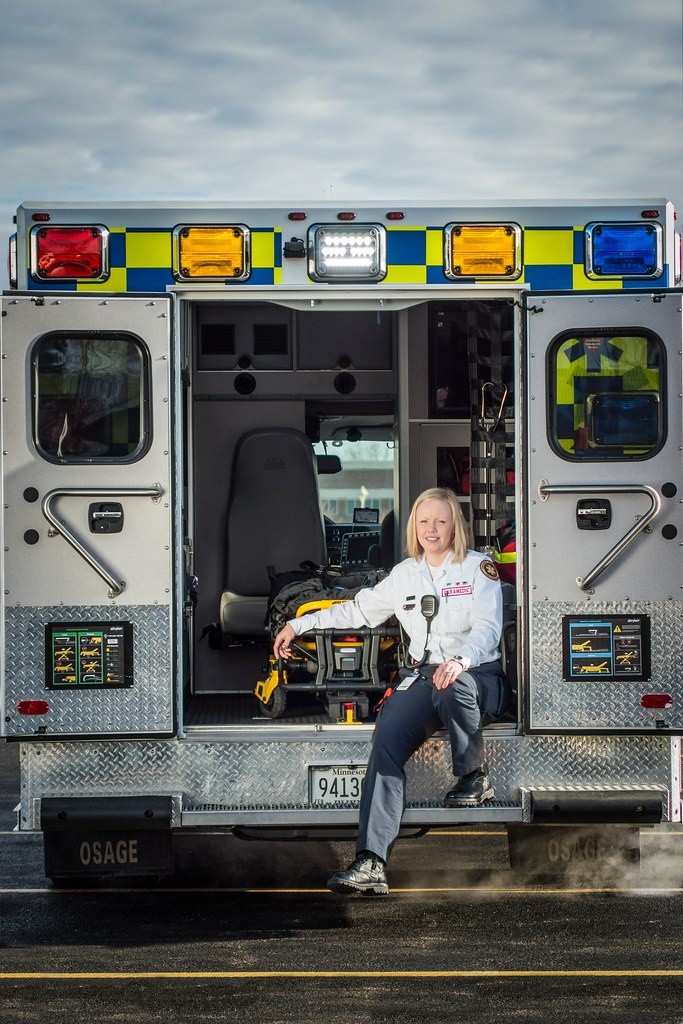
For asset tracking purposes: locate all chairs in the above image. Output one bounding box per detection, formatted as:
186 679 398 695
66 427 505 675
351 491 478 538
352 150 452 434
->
207 426 329 656
367 509 394 573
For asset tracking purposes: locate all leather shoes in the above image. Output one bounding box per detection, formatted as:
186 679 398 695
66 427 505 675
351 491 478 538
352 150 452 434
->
326 855 388 894
444 771 495 806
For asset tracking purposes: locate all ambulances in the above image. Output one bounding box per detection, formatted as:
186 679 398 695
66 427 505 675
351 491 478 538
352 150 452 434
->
1 195 683 883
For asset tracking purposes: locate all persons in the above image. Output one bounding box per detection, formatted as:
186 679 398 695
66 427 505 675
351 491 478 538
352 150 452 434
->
273 488 505 896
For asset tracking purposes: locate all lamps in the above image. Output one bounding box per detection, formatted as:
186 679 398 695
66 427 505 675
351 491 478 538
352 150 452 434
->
306 222 387 281
